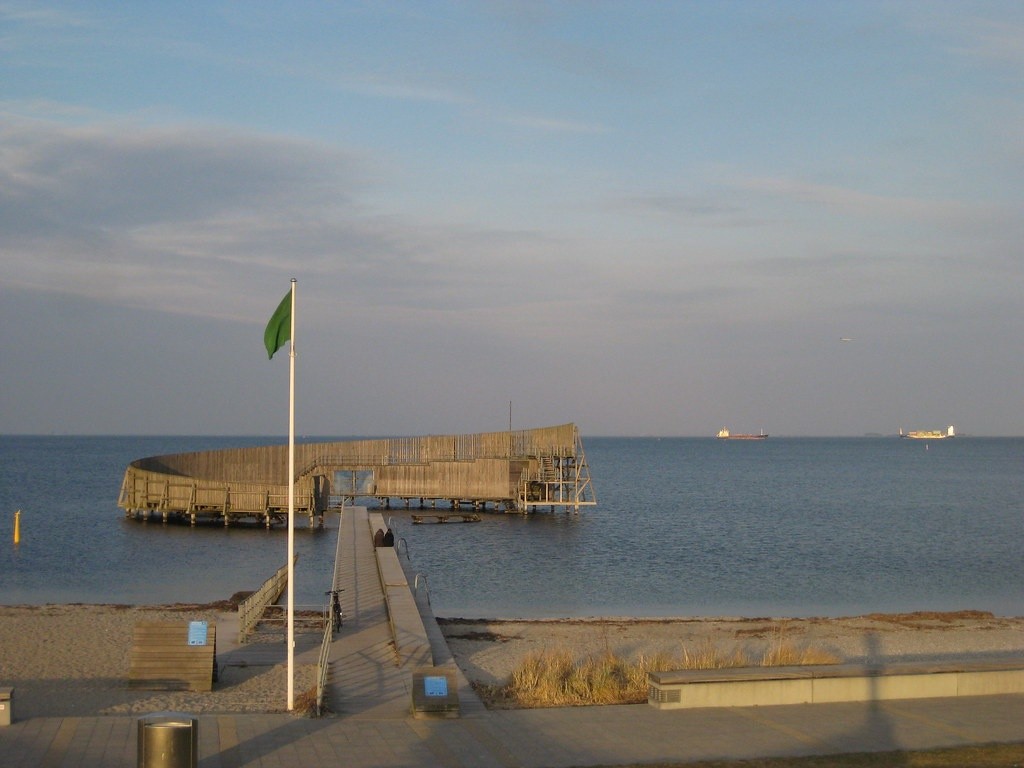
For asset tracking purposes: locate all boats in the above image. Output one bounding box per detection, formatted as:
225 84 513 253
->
898 425 956 440
715 425 768 440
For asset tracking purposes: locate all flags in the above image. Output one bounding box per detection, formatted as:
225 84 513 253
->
264 287 292 360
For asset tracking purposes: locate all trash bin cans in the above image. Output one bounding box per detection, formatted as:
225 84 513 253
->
137 717 198 768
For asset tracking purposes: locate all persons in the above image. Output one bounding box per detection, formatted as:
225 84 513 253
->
384 528 394 547
374 528 384 547
